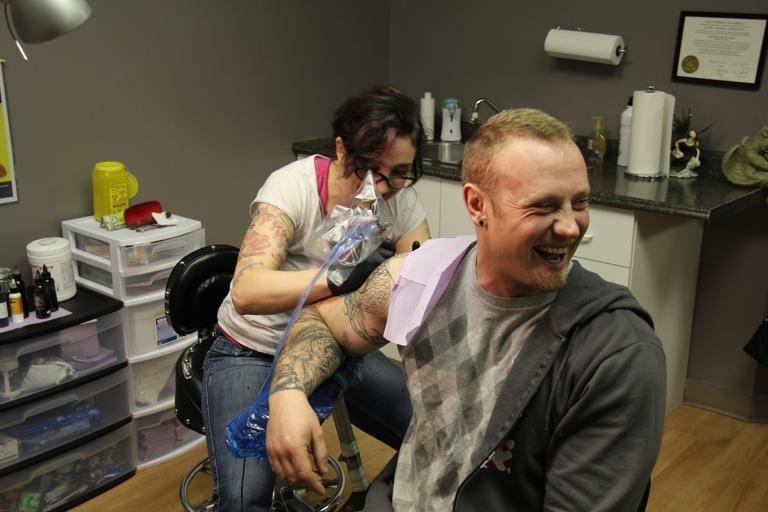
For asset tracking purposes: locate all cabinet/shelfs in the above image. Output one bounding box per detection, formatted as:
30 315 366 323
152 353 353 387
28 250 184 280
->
406 180 702 415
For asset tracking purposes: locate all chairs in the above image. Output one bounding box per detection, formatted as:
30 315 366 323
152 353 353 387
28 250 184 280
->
163 240 345 512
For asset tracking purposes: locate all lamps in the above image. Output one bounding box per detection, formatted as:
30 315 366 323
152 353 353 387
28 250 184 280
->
2 2 95 45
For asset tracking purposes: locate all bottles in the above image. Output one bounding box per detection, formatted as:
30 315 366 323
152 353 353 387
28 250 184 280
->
0 266 29 328
91 161 139 222
617 96 633 167
32 264 58 319
419 92 436 141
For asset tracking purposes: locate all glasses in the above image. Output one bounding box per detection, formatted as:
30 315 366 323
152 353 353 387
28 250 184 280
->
353 156 419 192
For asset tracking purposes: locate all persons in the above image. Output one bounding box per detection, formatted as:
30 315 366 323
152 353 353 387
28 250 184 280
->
201 84 433 512
264 106 663 512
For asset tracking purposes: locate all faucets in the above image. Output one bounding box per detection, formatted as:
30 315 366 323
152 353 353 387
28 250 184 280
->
469 98 501 124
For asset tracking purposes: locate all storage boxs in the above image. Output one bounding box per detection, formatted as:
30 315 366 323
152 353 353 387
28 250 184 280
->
62 209 208 472
1 281 138 512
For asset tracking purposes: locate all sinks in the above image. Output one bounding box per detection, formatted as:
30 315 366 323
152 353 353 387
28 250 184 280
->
416 139 468 166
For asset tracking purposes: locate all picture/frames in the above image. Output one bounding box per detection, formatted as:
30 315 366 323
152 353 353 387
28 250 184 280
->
671 10 768 90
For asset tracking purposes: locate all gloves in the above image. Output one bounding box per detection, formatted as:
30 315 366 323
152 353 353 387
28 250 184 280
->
326 235 396 297
